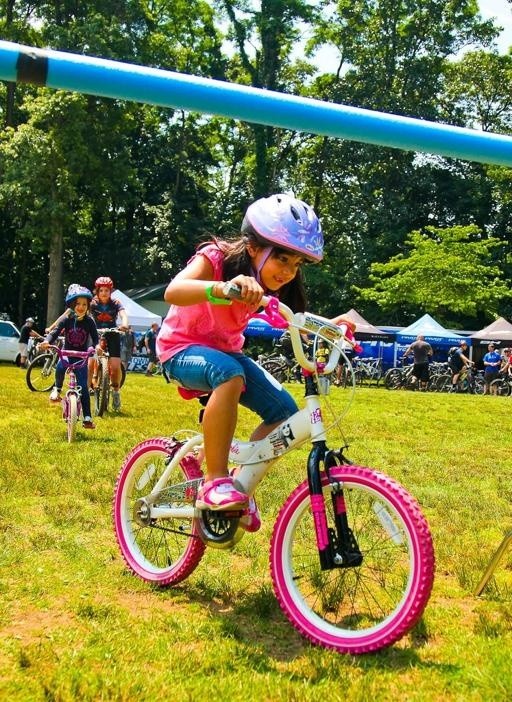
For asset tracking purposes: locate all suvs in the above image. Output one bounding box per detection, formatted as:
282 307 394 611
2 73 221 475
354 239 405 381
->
0 317 30 367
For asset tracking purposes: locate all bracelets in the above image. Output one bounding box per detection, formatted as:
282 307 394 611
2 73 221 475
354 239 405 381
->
208 279 220 297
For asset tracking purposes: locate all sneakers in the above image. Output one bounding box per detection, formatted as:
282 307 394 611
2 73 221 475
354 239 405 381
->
195 477 250 512
89 384 96 396
112 393 121 410
49 386 62 402
82 415 93 425
225 467 262 532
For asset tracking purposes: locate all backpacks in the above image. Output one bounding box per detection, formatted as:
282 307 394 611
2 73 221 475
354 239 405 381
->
447 345 462 363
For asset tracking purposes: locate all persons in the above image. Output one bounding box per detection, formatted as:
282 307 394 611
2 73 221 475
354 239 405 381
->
120 322 159 377
154 193 357 532
86 276 129 411
45 283 80 334
482 344 512 397
42 286 101 429
448 340 475 392
17 317 41 369
241 324 356 390
402 335 434 391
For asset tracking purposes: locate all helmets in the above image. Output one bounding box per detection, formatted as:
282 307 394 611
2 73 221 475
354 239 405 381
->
64 283 93 308
95 276 114 289
26 317 35 323
239 191 324 265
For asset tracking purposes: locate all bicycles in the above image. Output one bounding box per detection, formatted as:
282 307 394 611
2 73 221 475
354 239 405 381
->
21 326 136 444
110 276 439 659
252 349 512 396
